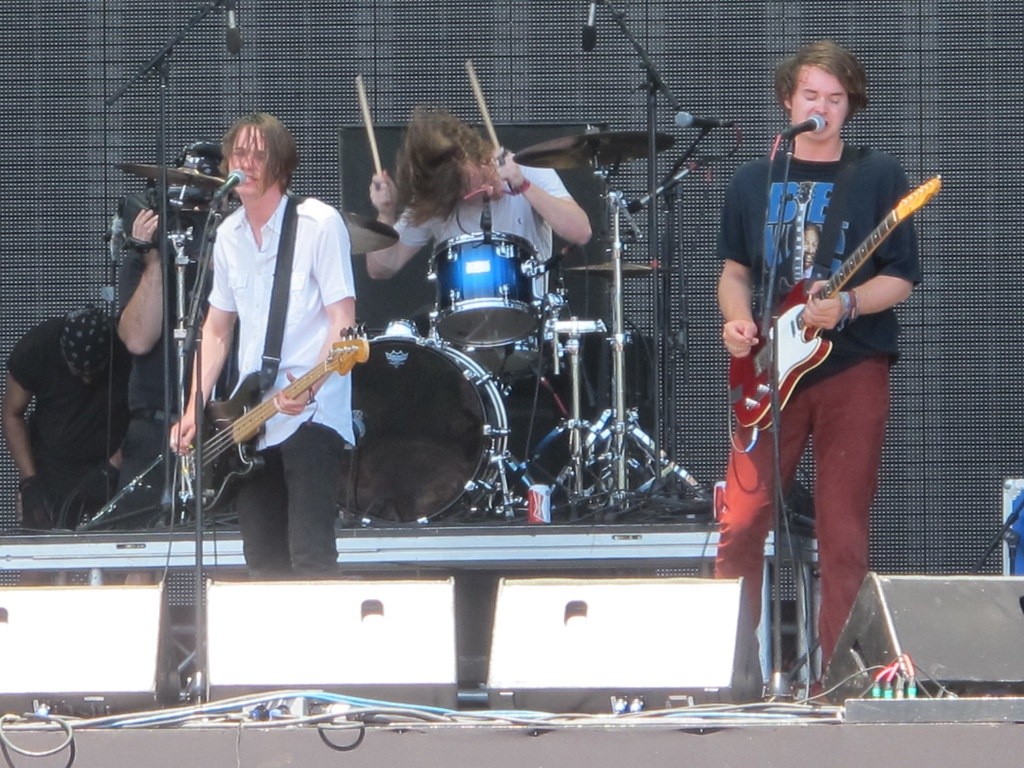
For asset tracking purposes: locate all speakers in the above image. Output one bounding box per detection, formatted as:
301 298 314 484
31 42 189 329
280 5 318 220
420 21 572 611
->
0 582 173 721
206 577 457 711
487 577 765 714
821 571 1024 706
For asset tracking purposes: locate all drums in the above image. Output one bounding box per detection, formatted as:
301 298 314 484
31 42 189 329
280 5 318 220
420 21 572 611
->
427 313 553 373
339 319 512 524
425 231 545 347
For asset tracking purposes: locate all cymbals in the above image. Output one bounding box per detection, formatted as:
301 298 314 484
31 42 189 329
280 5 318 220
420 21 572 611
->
340 209 400 255
512 130 678 169
112 161 226 188
563 260 664 271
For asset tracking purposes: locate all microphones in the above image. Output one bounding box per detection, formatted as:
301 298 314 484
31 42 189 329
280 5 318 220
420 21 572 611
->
780 115 825 139
212 169 246 201
225 0 243 54
582 0 597 51
627 161 699 213
675 111 733 128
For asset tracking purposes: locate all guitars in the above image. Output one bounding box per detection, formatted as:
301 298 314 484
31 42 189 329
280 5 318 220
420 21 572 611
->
727 173 946 430
178 333 373 518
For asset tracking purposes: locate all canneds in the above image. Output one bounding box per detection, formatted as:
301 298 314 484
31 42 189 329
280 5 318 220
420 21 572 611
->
527 484 551 525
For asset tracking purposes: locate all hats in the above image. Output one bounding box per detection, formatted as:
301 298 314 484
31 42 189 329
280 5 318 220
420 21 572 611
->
62 306 112 373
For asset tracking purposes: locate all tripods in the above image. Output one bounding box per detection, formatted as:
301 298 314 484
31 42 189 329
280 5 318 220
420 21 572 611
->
486 160 713 522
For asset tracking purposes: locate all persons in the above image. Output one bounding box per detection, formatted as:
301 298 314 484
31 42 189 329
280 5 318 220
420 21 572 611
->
710 42 924 725
368 108 593 353
117 139 241 530
170 113 357 579
2 302 133 529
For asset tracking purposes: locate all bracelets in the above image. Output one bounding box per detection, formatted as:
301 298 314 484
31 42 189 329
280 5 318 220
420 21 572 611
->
837 290 859 332
500 178 531 195
